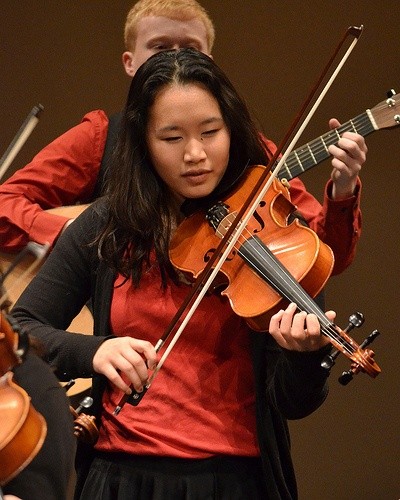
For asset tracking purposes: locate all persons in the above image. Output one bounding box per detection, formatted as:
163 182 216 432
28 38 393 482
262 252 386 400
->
0 0 367 276
8 48 336 500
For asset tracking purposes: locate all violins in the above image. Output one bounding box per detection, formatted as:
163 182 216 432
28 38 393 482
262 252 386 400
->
0 310 48 486
167 164 382 387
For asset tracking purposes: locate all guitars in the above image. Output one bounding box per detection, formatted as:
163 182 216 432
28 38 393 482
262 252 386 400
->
0 89 400 401
